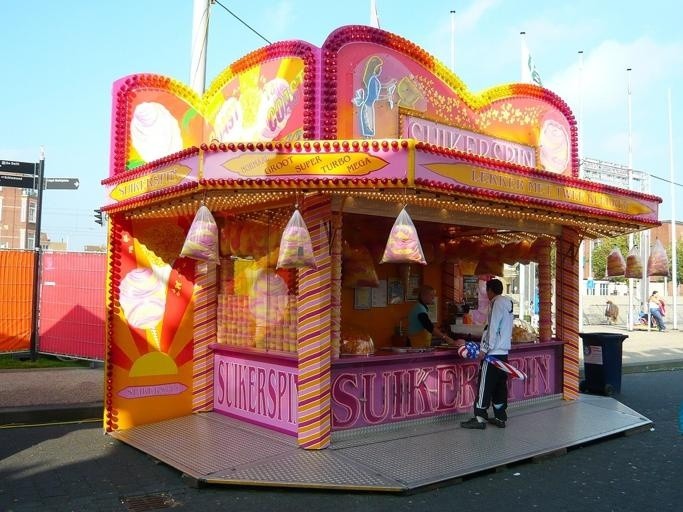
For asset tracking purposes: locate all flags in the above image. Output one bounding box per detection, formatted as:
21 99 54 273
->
457 342 527 382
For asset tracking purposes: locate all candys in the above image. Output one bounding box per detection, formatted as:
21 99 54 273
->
540 121 569 174
118 268 167 353
215 78 293 143
130 101 183 161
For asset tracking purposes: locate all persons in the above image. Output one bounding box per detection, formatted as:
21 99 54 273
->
459 277 512 430
405 284 457 349
641 289 666 332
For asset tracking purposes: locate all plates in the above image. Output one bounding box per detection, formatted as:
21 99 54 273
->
393 348 428 353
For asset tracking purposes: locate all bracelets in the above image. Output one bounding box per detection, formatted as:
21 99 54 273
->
440 334 446 340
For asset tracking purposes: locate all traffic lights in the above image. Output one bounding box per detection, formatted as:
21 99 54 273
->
93 209 102 227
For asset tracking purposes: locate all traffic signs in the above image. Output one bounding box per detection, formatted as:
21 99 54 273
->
43 177 78 190
0 159 38 175
0 175 38 190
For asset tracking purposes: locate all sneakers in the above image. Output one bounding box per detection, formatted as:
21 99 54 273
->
488 417 506 428
461 418 485 429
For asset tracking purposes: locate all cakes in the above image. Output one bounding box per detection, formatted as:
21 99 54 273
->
341 339 371 354
511 327 532 341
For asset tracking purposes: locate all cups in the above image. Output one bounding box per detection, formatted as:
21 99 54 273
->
455 312 473 325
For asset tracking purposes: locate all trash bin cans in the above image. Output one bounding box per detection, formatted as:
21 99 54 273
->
578 332 628 396
